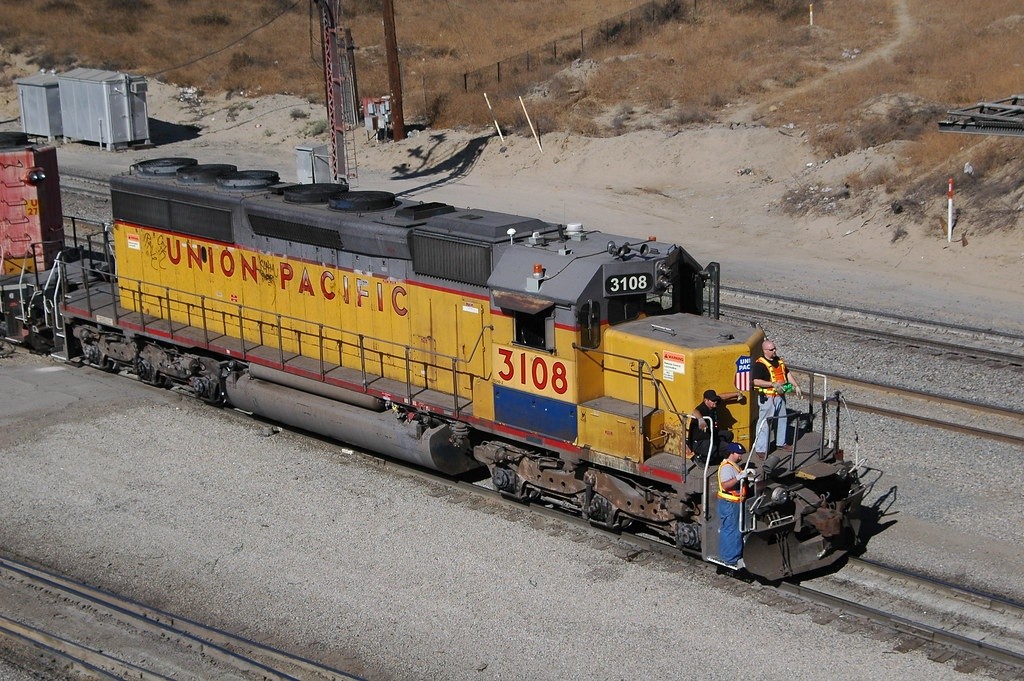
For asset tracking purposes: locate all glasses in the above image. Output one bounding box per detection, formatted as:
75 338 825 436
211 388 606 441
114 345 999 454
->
764 347 776 351
708 399 716 402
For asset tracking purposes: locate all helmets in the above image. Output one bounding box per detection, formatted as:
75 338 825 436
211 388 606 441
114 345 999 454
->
727 443 746 454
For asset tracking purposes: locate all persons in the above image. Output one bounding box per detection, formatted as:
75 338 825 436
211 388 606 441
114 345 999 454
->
717 442 748 568
751 339 803 461
688 389 745 470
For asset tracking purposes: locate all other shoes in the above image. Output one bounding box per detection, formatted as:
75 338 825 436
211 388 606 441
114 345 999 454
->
776 444 793 451
718 558 737 566
756 452 766 460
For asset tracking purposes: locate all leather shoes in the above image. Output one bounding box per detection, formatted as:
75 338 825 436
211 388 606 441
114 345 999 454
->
690 455 705 470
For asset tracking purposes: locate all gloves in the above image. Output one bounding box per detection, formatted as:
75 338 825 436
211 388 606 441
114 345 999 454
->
795 385 803 401
737 392 743 401
736 471 747 480
698 418 708 432
774 383 785 395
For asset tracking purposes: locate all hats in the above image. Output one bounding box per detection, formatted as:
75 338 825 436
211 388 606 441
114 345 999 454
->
703 390 719 401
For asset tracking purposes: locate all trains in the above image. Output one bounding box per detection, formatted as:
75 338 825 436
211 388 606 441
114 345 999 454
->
0 143 900 582
